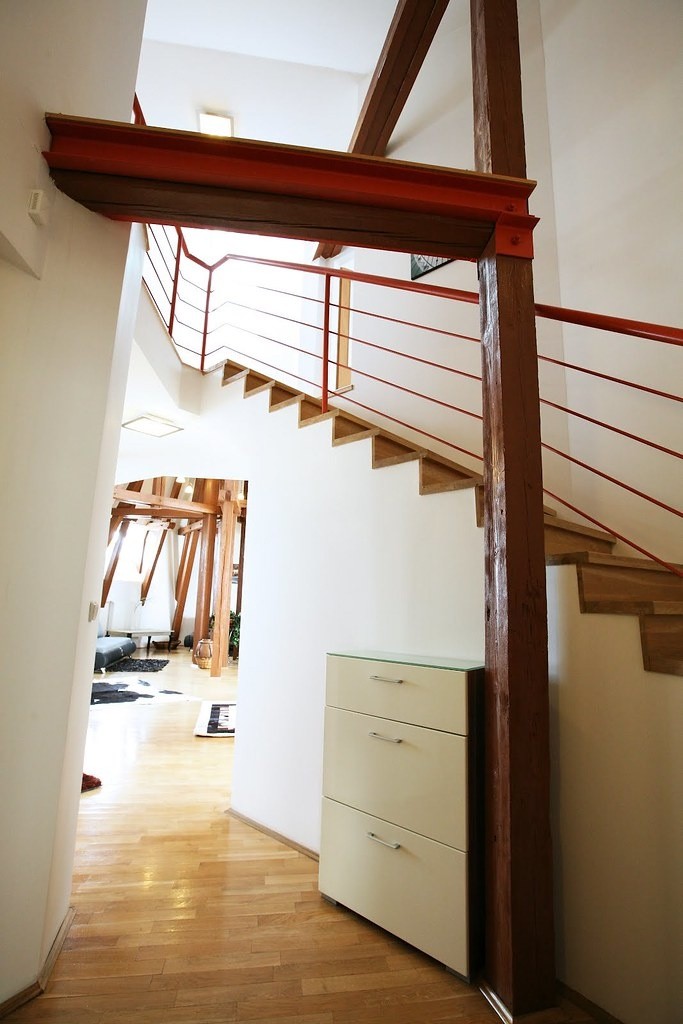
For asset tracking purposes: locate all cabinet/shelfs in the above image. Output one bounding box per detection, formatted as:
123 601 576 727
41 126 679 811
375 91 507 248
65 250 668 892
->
317 649 487 984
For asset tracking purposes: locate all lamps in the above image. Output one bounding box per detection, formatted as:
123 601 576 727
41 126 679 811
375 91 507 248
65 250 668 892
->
120 413 185 439
198 110 235 137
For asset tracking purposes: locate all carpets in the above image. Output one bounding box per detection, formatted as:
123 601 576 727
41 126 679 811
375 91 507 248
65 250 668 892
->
104 658 169 672
191 700 236 738
81 773 101 793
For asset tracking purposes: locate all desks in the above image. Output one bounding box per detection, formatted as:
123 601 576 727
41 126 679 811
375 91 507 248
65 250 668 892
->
106 628 175 652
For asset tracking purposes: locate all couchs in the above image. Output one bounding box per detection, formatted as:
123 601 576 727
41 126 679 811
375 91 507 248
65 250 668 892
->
94 624 136 673
